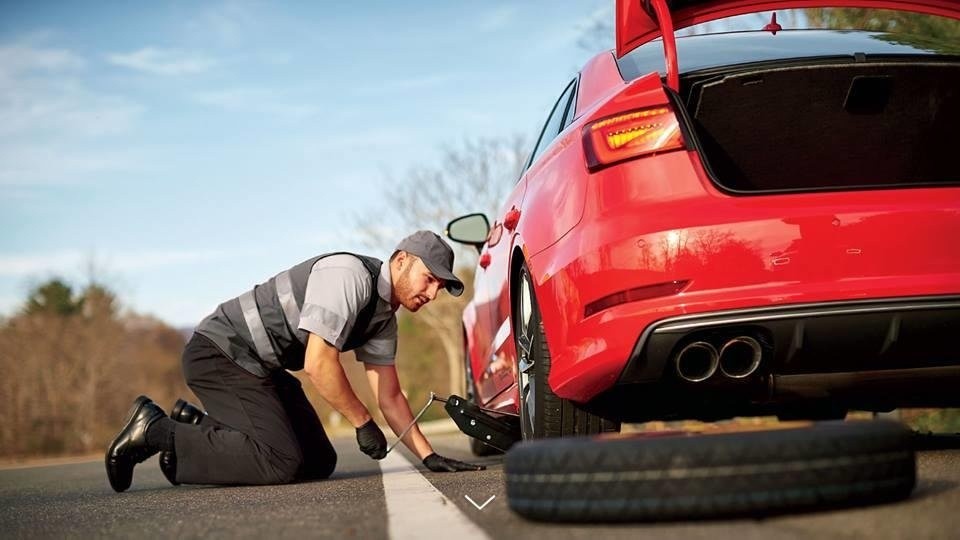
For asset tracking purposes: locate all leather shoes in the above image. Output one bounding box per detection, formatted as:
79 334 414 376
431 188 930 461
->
104 395 168 493
159 398 204 485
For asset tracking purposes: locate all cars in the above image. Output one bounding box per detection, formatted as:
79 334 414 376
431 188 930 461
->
444 1 960 469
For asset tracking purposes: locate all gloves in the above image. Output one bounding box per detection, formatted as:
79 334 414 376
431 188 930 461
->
422 452 487 472
354 417 387 460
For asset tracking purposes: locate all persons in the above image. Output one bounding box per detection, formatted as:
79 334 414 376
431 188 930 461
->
104 230 487 494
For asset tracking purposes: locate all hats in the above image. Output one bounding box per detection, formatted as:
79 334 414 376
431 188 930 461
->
395 230 464 297
